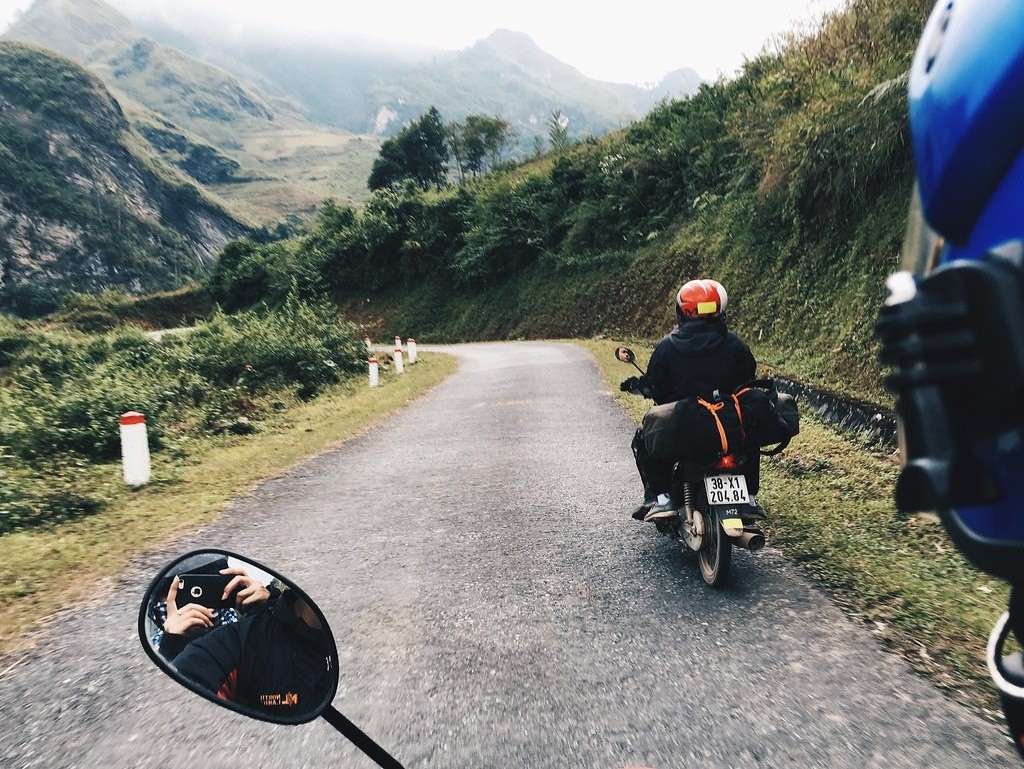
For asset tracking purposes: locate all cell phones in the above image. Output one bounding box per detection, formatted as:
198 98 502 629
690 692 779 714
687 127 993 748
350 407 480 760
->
176 574 247 610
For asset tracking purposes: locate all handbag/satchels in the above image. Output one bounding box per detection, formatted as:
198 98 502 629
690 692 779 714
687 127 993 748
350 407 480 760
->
673 379 791 456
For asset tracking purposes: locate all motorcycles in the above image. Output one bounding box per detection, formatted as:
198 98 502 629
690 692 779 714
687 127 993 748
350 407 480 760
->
615 344 766 589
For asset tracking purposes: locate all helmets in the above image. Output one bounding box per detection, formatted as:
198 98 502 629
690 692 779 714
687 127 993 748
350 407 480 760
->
154 553 228 606
876 0 1024 576
702 279 728 314
676 280 724 322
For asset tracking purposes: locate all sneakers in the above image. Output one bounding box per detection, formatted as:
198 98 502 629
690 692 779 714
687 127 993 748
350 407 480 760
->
643 501 679 522
739 501 768 520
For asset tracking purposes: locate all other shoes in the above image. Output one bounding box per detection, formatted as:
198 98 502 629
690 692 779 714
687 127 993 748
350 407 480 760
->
632 506 651 518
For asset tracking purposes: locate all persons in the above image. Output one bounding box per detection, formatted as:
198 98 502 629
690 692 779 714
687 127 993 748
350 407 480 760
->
618 348 630 361
153 555 328 720
871 1 1024 769
617 279 758 524
